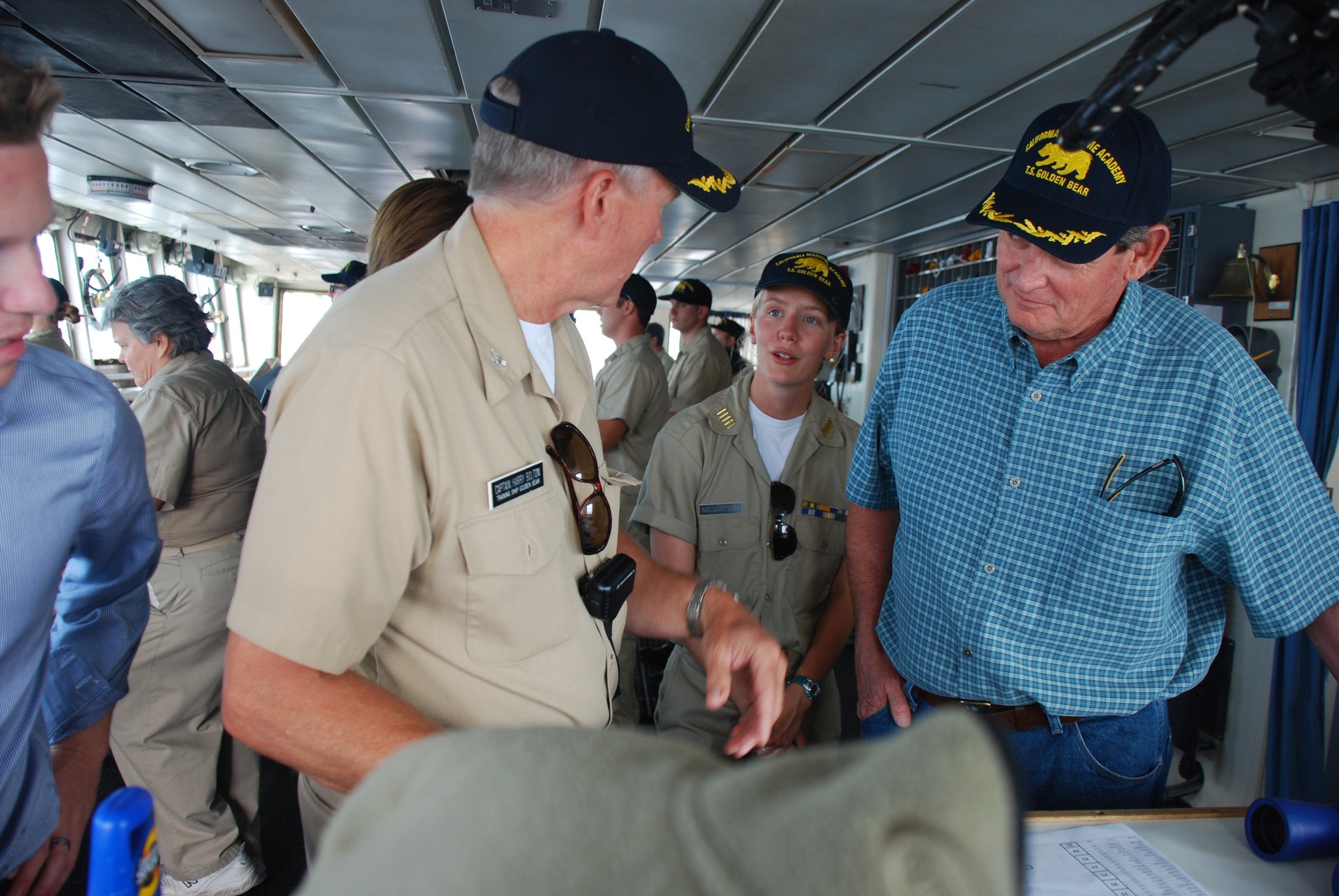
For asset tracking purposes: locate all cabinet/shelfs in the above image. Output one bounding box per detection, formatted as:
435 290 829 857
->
892 202 1256 345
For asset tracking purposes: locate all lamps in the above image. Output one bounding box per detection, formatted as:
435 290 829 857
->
1211 241 1303 322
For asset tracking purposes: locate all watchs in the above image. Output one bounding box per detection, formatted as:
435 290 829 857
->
786 674 822 701
684 577 741 638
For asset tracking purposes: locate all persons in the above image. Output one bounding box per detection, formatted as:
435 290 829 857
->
644 322 675 379
22 274 81 361
627 250 863 761
322 260 368 302
707 317 754 387
366 177 473 277
219 25 746 888
656 277 733 420
842 94 1338 814
104 274 267 896
594 271 670 556
1 54 166 895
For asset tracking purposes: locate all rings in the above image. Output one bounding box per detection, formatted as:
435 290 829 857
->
48 835 72 850
759 747 770 757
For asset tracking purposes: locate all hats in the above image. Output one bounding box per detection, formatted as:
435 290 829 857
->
965 92 1172 265
322 260 367 288
657 278 712 306
44 276 71 312
710 318 746 343
479 28 742 213
646 323 665 339
753 251 854 331
620 273 657 315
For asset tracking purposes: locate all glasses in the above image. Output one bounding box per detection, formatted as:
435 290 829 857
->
545 421 613 555
1100 452 1185 518
770 481 798 561
329 285 346 293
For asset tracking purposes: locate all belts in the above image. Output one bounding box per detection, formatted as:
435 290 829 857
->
902 678 1087 735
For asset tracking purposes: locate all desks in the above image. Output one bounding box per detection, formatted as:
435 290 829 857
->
1020 806 1339 895
